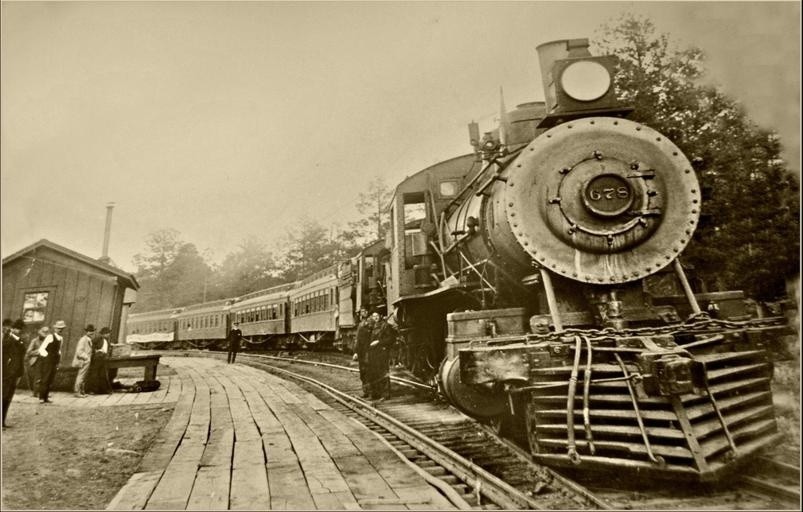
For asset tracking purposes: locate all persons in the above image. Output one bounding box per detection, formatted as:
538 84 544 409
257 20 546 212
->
350 305 373 399
1 317 112 431
365 311 395 400
226 322 242 364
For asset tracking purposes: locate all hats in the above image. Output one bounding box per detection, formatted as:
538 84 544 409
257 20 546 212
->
11 318 26 329
84 323 96 333
52 320 67 329
37 326 51 338
98 327 112 335
232 322 239 326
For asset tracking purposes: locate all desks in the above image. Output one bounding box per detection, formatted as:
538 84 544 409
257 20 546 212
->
104 353 164 382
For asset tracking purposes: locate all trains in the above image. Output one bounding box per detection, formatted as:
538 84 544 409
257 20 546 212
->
122 36 780 486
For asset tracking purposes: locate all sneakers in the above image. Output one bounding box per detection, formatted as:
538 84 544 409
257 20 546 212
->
33 392 90 404
